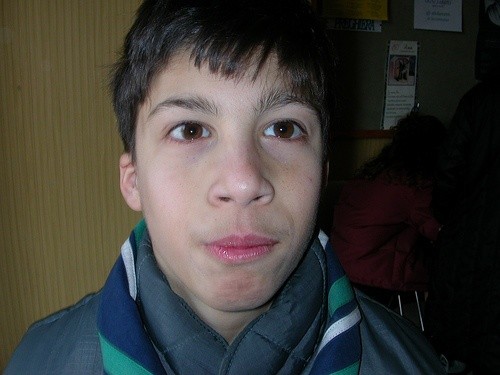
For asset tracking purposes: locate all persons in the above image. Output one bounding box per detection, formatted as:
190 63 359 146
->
329 110 469 372
3 1 448 375
